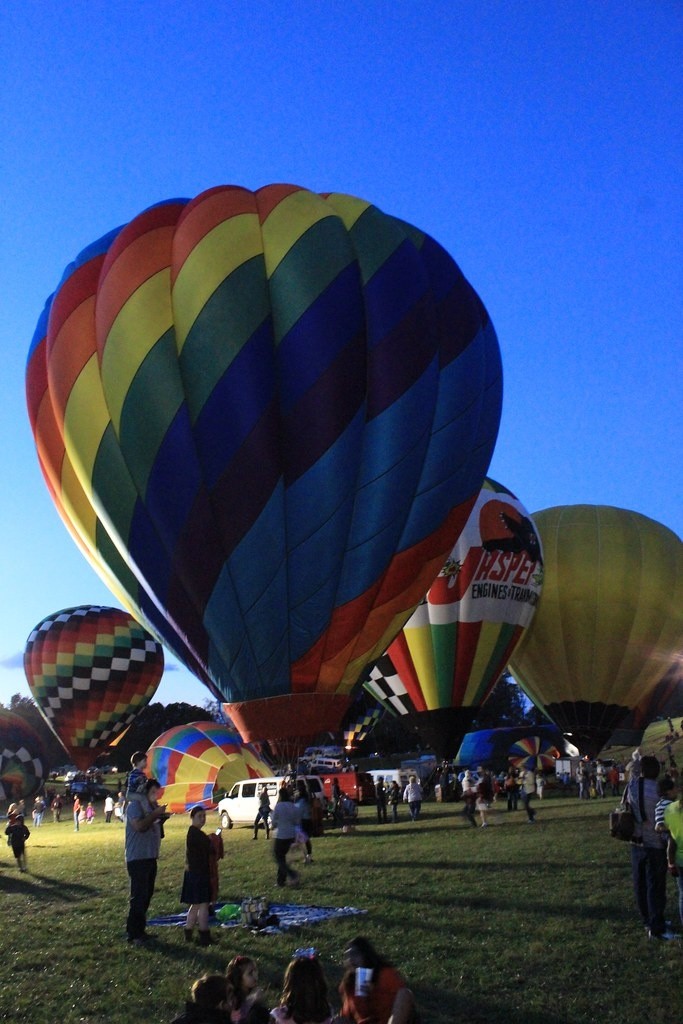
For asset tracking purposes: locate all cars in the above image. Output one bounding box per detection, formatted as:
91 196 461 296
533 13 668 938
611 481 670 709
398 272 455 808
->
70 781 109 804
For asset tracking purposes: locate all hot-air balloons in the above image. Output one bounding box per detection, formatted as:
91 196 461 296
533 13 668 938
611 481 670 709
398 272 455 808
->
144 720 275 816
26 182 507 794
503 503 683 767
508 737 560 776
360 474 544 802
0 707 50 806
24 604 163 774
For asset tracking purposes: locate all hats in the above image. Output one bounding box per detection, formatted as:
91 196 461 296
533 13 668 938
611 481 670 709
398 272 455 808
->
190 806 203 818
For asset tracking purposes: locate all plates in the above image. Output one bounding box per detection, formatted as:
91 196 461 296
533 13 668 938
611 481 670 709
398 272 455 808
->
158 813 172 818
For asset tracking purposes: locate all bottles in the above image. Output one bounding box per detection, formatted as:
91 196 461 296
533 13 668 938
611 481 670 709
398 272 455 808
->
250 900 259 929
242 897 250 929
259 897 269 918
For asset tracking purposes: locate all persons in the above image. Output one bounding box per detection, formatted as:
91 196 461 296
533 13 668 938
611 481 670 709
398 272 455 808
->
253 761 359 839
607 745 683 938
6 777 128 874
272 788 303 891
170 938 415 1024
576 753 606 800
522 764 536 824
126 751 167 944
182 806 220 947
375 775 423 824
434 762 547 829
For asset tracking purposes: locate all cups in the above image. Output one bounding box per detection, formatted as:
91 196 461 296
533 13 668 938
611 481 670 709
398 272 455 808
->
355 966 373 997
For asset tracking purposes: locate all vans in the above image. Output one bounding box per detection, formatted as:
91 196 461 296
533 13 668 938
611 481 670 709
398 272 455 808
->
317 772 375 805
366 768 421 796
307 757 343 774
217 773 329 829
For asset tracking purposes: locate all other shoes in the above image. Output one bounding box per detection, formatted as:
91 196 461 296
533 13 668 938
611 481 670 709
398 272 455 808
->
527 818 537 825
288 871 301 888
274 883 285 887
74 829 79 832
303 854 313 863
134 934 158 951
18 867 22 873
531 810 538 820
468 823 477 830
21 867 26 873
616 794 623 797
613 794 617 796
480 823 490 829
411 818 420 822
126 934 159 944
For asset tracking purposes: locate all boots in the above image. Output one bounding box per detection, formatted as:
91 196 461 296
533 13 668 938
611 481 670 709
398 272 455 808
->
182 928 194 942
196 930 219 948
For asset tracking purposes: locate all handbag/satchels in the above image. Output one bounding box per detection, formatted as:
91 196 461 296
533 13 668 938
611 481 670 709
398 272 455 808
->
609 809 636 843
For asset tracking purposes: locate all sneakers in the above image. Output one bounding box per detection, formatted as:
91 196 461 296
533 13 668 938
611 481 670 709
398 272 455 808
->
648 929 680 944
644 920 672 933
